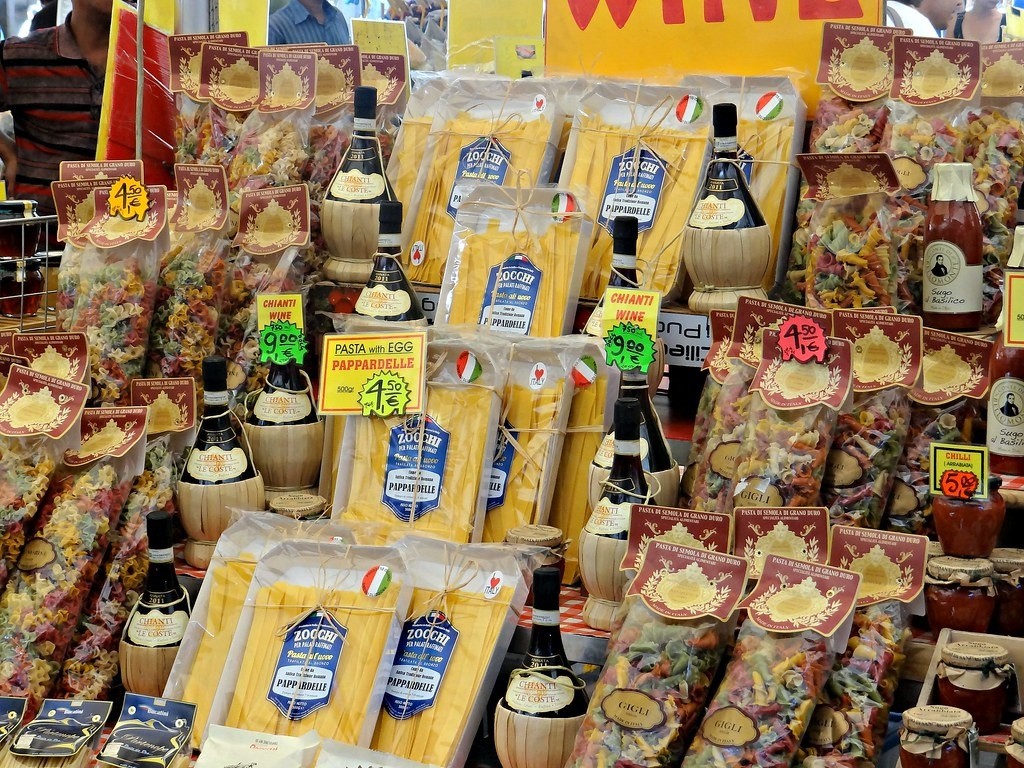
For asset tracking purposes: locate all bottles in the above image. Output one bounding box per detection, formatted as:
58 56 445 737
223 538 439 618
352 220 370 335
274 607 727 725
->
321 87 397 204
986 299 1024 475
923 162 982 331
589 368 679 474
119 512 191 650
579 397 657 539
351 202 428 321
682 104 771 230
580 217 665 338
494 567 590 718
177 356 264 484
241 357 323 427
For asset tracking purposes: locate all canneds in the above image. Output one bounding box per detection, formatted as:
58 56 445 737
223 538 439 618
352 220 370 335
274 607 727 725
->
924 556 997 640
937 642 1011 736
907 541 945 631
899 705 973 768
989 547 1024 638
36 254 63 312
505 525 566 606
1004 717 1024 768
934 473 1005 558
0 200 41 260
268 494 326 520
0 260 45 317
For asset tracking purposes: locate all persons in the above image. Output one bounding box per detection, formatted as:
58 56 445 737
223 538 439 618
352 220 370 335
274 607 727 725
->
268 0 352 46
0 0 137 268
